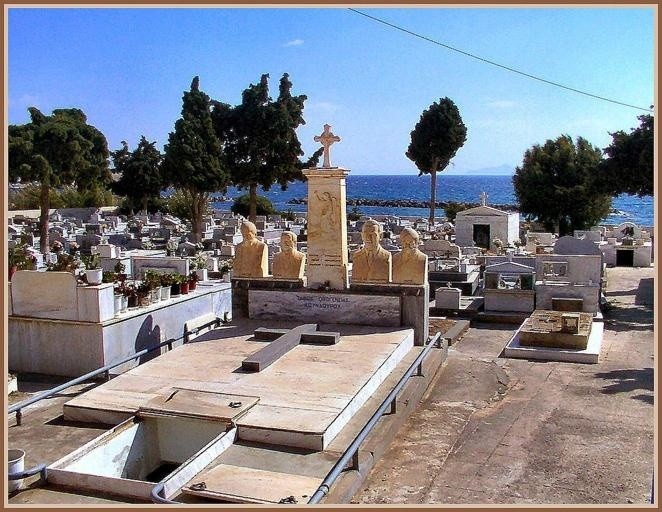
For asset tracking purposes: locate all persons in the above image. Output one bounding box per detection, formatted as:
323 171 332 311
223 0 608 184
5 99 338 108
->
352 220 392 282
233 222 268 278
392 227 429 284
273 230 306 279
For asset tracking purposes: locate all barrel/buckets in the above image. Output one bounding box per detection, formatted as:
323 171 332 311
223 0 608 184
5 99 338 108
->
8 448 26 492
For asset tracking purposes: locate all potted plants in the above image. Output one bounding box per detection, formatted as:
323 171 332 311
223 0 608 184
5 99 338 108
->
8 237 198 316
